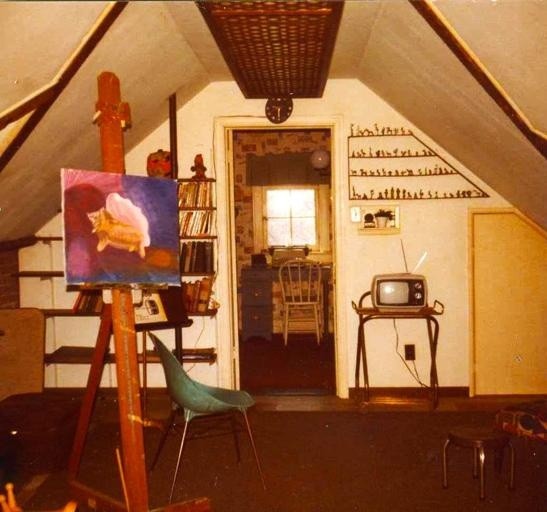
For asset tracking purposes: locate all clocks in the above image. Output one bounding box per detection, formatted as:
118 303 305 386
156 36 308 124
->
265 95 294 124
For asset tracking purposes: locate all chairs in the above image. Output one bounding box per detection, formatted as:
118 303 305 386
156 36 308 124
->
147 333 267 505
279 260 322 347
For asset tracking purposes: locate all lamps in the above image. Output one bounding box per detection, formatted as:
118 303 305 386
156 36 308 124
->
310 150 329 171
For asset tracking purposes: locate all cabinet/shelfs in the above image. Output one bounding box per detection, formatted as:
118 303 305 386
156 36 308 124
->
352 292 441 412
242 270 273 341
9 176 216 366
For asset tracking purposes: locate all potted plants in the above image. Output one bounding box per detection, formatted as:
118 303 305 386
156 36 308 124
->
375 209 393 229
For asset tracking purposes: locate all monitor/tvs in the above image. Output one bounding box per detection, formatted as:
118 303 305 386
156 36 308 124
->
371 274 428 312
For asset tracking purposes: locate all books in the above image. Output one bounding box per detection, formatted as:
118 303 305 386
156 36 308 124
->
72 290 104 313
179 241 214 274
181 277 212 313
177 209 218 237
178 181 211 208
172 348 215 359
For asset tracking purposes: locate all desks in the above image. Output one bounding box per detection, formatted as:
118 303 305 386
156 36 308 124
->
241 264 330 341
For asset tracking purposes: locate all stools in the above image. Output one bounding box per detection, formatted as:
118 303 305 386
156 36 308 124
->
494 401 547 474
441 423 515 501
